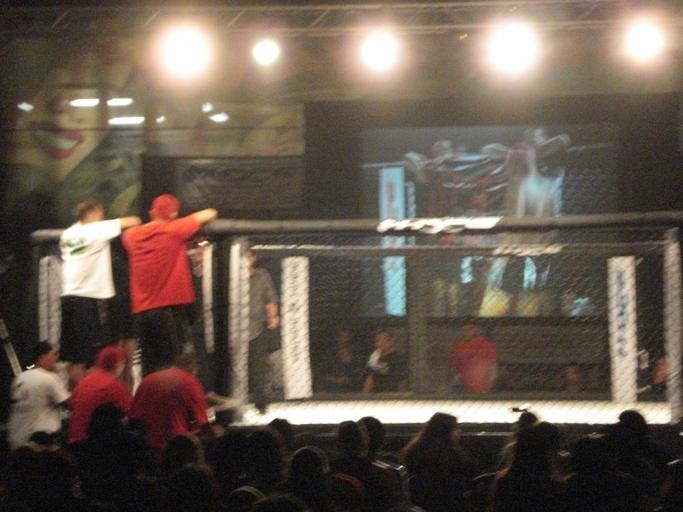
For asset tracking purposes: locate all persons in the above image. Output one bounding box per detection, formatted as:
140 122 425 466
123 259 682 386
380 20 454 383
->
314 327 371 397
126 336 224 453
629 356 672 402
4 339 81 459
242 247 286 415
0 34 143 199
1 400 681 512
558 361 592 399
396 137 491 319
349 326 413 399
444 319 501 399
64 342 140 444
58 195 145 362
185 237 230 391
120 192 221 374
475 127 573 323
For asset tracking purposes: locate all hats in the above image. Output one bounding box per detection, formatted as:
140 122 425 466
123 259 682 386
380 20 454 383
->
26 339 52 368
96 345 126 370
149 193 181 216
